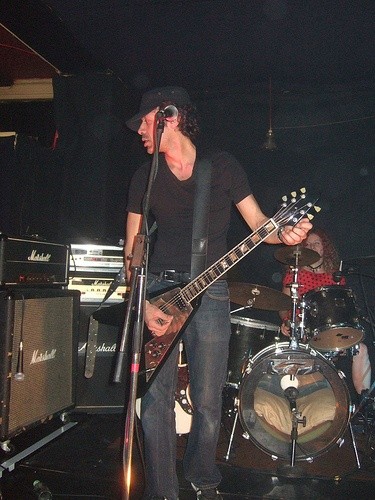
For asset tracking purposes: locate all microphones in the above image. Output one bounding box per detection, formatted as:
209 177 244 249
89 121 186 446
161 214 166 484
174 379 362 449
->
333 261 343 282
283 387 298 411
156 105 178 122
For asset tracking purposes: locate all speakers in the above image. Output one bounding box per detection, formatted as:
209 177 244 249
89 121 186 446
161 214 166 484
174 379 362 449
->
0 286 132 441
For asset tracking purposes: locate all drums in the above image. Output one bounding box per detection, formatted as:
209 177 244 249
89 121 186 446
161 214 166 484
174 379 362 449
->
238 341 355 464
303 285 366 353
223 315 281 390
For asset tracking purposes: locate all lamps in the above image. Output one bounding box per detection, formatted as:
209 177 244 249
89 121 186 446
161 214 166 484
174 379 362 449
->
264 74 278 153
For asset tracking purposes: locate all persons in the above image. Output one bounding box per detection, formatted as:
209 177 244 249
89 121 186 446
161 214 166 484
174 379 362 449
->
280 230 375 421
124 87 313 500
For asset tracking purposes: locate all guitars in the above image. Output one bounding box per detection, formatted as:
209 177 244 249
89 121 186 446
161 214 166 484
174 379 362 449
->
93 187 321 399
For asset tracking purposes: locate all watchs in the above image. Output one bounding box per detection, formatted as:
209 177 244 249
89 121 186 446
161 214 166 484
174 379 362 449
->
278 226 284 243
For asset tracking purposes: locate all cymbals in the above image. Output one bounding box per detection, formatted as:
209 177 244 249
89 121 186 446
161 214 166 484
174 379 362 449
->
227 281 294 311
273 245 320 266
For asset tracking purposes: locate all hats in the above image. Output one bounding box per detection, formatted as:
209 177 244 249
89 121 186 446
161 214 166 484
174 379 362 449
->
124 86 190 131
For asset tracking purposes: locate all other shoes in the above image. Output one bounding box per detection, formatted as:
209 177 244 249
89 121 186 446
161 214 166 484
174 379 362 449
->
139 492 178 500
189 482 223 500
358 390 375 415
